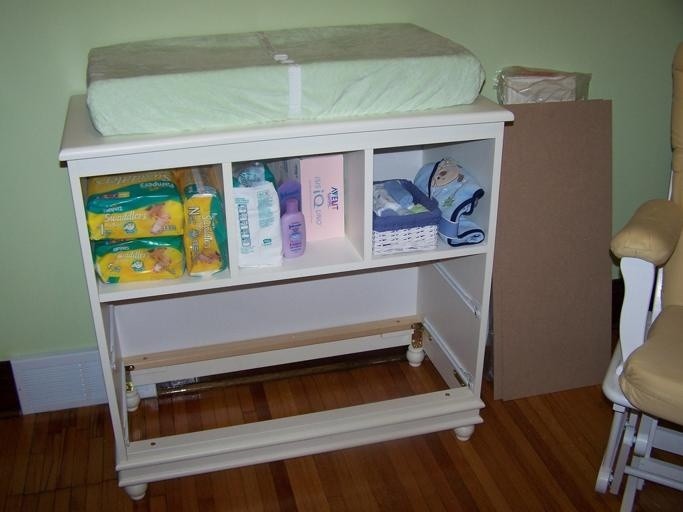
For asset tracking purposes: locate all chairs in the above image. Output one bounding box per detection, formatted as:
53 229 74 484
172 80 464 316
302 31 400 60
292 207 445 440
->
591 37 683 512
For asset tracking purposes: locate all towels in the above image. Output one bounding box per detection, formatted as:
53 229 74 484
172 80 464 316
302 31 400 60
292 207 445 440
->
385 180 413 207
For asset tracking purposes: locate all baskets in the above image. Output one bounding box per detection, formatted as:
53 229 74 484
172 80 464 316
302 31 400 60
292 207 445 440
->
372 180 441 255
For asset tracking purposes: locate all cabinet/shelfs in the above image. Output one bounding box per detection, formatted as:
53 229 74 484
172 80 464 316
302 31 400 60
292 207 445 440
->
58 92 515 500
